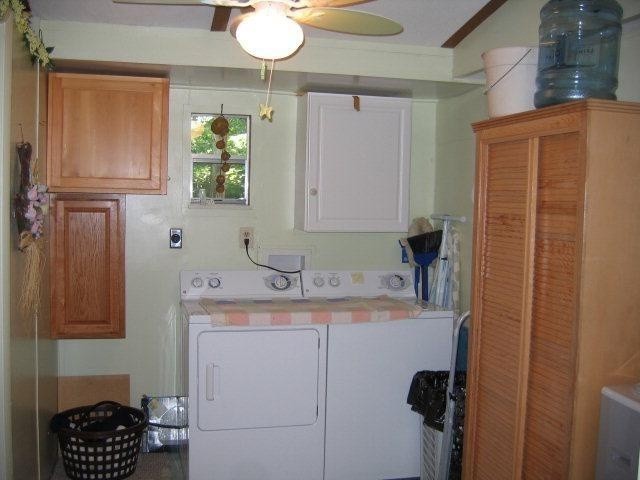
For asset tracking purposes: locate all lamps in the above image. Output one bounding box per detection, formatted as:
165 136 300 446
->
235 11 304 60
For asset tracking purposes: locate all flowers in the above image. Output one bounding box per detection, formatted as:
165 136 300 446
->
25 183 56 239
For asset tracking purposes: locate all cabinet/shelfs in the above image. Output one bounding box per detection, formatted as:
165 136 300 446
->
47 72 169 195
460 97 639 480
294 92 412 233
49 192 125 339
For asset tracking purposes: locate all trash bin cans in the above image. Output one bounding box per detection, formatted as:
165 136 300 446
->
417 370 450 480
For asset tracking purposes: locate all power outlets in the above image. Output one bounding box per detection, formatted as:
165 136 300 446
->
239 227 255 249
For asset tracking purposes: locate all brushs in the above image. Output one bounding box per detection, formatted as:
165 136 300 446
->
406 230 442 303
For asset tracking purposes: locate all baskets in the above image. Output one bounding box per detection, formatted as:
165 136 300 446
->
51 401 147 480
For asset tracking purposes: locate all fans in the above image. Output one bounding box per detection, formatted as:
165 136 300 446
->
113 0 404 36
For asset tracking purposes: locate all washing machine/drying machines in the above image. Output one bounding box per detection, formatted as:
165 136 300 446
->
178 267 330 479
298 269 455 479
